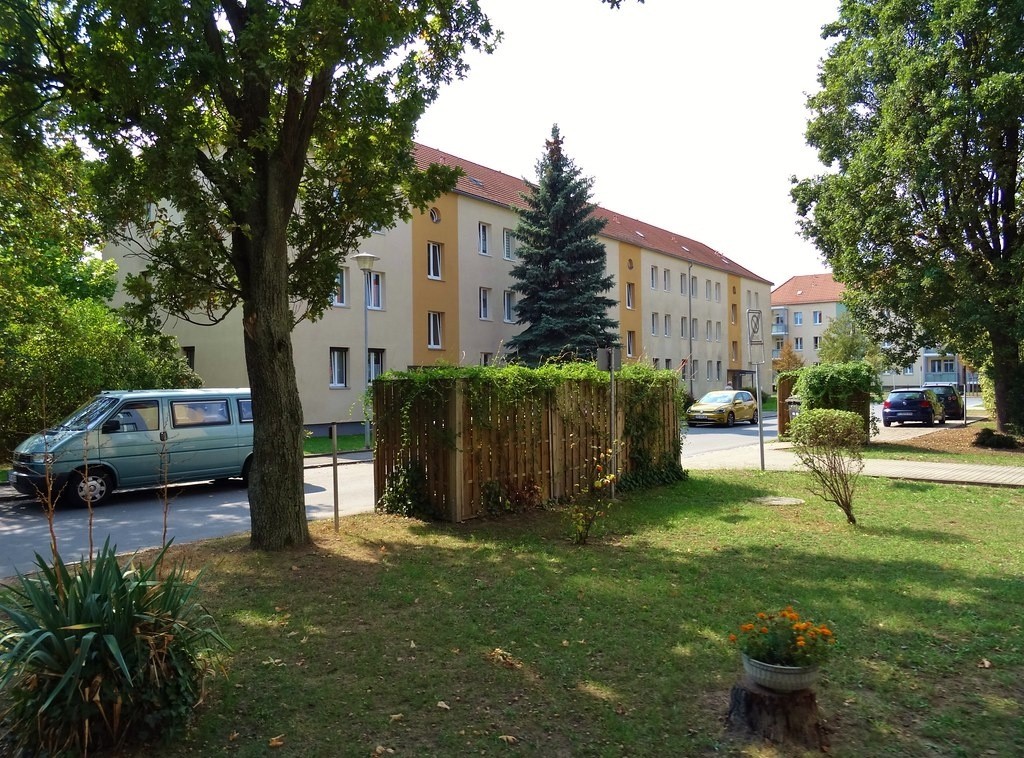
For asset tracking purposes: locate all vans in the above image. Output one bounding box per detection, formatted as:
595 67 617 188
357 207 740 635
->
9 388 254 508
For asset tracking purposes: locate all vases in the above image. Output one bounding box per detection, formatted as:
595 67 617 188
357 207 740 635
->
742 653 817 691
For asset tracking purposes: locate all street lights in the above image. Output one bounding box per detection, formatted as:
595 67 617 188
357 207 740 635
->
349 252 380 450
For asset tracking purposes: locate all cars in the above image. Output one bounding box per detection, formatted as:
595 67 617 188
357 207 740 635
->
921 382 965 420
686 390 758 427
882 388 944 427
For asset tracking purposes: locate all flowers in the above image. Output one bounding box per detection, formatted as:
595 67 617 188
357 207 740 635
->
729 605 835 670
